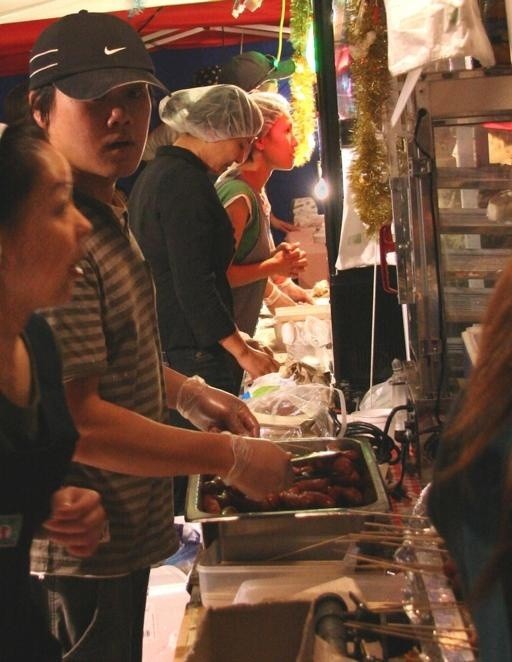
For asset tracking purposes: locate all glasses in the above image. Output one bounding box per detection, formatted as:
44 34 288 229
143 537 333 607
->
264 54 278 77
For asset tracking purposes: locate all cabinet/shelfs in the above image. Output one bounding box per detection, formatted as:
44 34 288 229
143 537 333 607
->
387 72 512 416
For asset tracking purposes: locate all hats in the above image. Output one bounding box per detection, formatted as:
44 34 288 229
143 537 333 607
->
193 65 224 87
29 10 171 102
227 51 295 94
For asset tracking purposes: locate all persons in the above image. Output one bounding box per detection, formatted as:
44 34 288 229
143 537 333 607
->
1 123 111 659
130 84 281 568
214 91 307 336
23 9 295 660
1 50 322 323
427 260 512 662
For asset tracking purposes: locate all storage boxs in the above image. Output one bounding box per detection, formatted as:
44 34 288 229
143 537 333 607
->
140 565 191 661
